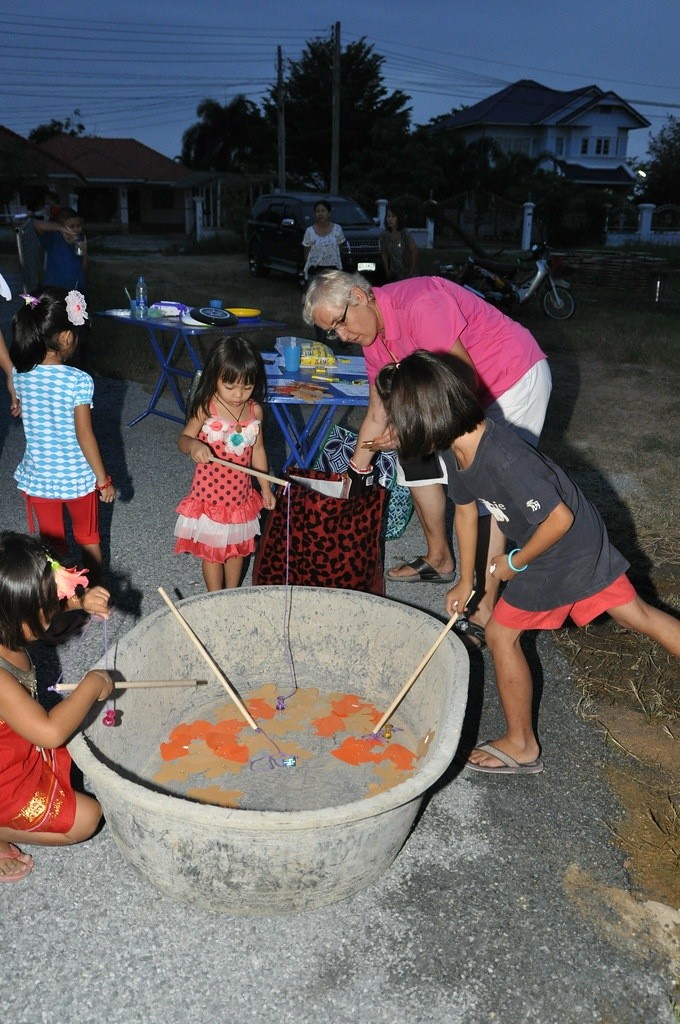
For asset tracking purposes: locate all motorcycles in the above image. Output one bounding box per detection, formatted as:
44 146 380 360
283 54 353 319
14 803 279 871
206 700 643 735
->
431 238 576 322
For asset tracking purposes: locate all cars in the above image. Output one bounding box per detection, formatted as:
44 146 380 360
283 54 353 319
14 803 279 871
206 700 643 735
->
19 206 96 260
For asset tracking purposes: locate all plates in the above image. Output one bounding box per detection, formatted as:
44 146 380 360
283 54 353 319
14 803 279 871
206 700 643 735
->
181 307 261 327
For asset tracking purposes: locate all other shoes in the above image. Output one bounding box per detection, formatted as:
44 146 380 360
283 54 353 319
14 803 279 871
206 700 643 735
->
337 340 351 349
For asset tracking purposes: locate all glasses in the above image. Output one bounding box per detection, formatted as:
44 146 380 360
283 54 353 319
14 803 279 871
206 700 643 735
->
326 291 352 341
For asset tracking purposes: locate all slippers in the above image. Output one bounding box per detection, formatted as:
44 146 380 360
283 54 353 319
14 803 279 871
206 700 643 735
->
455 739 544 774
383 557 455 583
452 617 488 656
43 613 90 645
90 596 119 621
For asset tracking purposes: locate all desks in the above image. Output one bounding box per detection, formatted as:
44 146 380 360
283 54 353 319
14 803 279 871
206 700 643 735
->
257 352 370 472
97 308 289 429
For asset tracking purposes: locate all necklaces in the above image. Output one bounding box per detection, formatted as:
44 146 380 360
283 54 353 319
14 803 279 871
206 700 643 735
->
214 393 246 432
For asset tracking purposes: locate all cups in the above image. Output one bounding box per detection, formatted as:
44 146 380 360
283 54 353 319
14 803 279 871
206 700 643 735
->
210 300 222 310
284 346 301 373
130 299 137 315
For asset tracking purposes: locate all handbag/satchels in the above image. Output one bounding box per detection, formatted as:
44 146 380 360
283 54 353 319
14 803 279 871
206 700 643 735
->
313 424 413 540
274 336 336 368
252 466 384 597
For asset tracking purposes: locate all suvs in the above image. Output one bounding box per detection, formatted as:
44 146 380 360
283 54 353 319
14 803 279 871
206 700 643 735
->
243 189 389 292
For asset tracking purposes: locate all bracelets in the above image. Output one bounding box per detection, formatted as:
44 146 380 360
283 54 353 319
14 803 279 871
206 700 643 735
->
508 548 528 572
96 475 112 490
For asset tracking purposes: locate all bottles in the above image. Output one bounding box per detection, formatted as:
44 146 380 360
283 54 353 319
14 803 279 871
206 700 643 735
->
135 276 149 321
73 236 85 256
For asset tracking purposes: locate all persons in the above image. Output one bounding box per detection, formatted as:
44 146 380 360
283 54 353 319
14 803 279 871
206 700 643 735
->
374 349 680 776
379 203 416 281
301 199 354 348
0 528 114 882
0 269 116 564
36 205 89 298
173 334 276 592
33 191 64 220
303 269 552 651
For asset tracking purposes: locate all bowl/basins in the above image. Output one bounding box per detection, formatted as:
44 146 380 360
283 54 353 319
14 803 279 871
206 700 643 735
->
66 585 469 917
